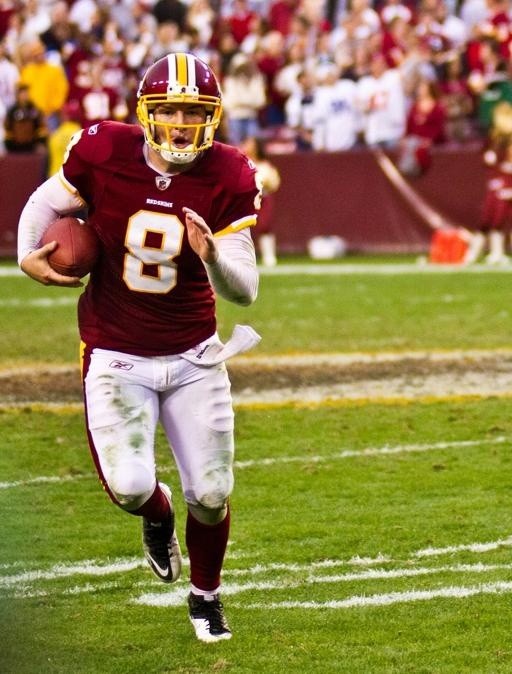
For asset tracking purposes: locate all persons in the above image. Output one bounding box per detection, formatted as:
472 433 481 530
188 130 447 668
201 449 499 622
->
238 137 280 267
17 54 261 647
462 101 512 264
0 0 512 176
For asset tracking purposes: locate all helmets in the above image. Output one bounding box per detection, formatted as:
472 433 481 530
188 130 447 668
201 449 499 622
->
137 52 222 163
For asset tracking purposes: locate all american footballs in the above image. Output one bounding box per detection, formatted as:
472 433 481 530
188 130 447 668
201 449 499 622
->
40 218 99 279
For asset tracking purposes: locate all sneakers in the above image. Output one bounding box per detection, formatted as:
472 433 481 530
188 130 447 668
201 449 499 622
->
188 592 232 643
142 482 182 583
